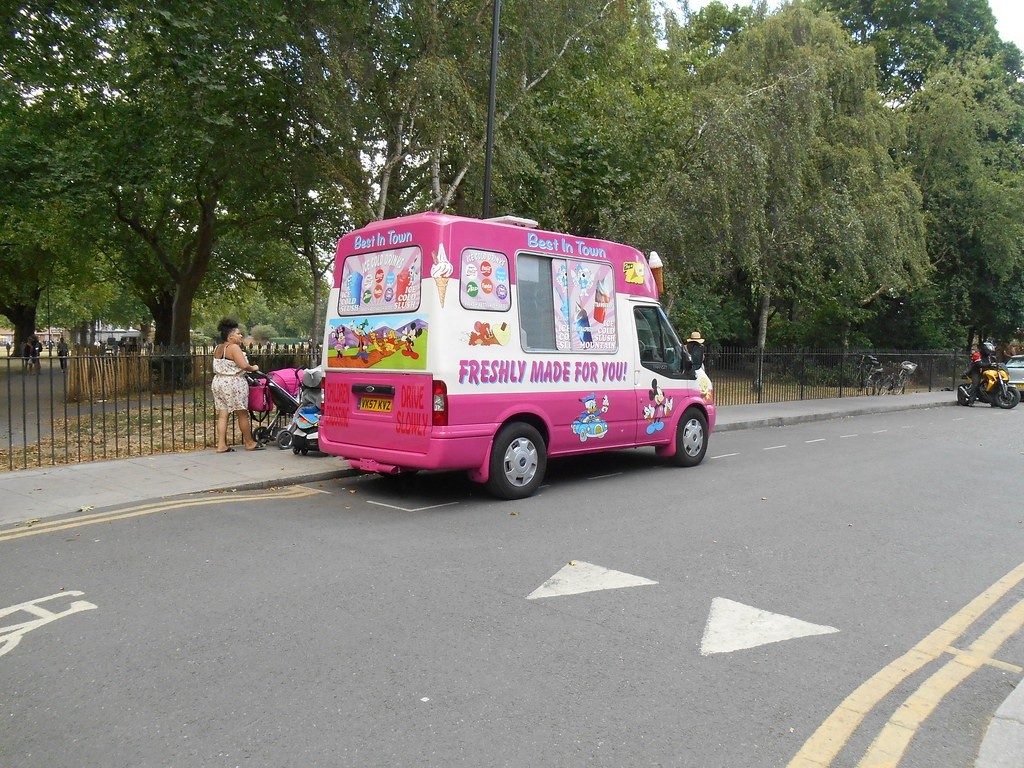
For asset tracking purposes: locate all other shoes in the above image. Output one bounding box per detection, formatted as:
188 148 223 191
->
969 403 976 407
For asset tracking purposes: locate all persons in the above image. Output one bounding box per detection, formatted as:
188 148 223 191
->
22 335 43 375
686 332 705 370
211 318 266 453
57 338 69 373
118 337 149 353
966 342 997 407
1004 344 1014 363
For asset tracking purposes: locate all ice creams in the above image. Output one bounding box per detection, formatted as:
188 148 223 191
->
430 243 453 308
574 301 587 322
648 251 663 293
596 276 610 297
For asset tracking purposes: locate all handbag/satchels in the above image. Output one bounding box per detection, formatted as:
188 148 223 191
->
248 385 273 422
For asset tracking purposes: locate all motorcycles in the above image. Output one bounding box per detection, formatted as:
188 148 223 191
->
958 355 1022 409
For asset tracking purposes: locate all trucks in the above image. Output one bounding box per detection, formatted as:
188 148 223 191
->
319 212 718 501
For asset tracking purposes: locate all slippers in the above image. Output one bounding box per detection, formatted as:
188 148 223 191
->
245 442 266 450
217 447 237 453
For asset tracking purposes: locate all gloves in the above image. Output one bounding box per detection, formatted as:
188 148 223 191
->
990 362 997 368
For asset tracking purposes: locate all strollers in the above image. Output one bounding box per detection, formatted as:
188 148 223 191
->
245 368 305 450
291 365 322 456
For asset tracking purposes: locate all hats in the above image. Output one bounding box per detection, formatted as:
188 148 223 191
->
687 332 705 344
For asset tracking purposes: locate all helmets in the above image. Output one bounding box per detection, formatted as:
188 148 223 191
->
981 342 995 355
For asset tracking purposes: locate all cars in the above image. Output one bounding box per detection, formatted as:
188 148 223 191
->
1005 355 1024 402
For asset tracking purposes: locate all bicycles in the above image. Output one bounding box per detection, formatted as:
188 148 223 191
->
855 354 918 396
